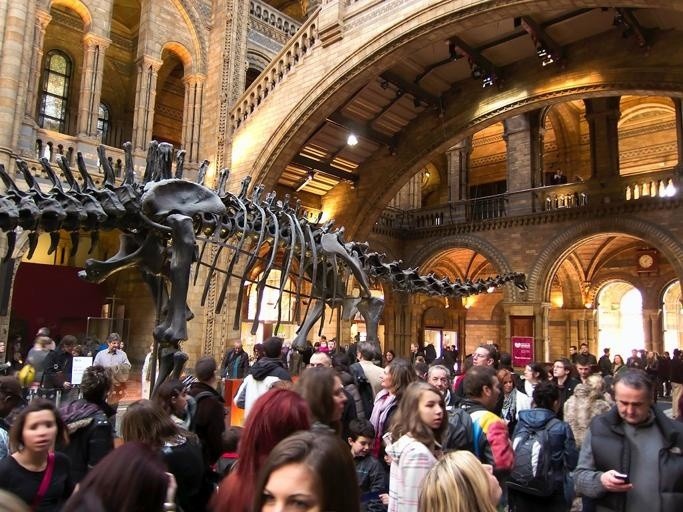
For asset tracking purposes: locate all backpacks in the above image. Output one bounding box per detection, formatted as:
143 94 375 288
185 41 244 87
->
442 403 494 452
185 391 213 418
505 418 561 497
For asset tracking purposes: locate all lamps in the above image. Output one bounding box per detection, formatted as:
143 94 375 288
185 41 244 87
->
447 44 483 80
611 12 633 39
378 77 437 116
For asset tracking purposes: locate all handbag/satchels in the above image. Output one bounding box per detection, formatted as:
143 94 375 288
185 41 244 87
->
37 386 62 408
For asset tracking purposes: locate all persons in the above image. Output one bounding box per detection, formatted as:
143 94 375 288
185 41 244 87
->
550 167 567 185
572 175 588 206
0 323 682 512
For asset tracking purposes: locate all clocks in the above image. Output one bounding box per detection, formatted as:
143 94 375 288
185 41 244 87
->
635 248 659 277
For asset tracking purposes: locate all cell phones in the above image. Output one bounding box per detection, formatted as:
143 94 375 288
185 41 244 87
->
614 474 630 484
